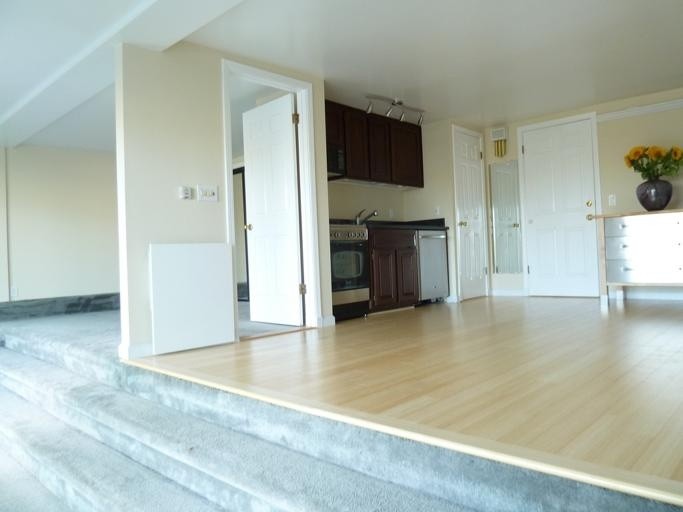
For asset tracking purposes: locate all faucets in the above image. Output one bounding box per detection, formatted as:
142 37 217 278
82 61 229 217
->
355 208 365 224
359 210 379 226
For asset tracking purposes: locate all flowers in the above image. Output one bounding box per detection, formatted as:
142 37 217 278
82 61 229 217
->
622 142 683 180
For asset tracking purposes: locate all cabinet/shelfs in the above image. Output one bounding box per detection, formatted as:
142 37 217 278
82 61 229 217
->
344 107 392 185
585 208 682 313
393 118 424 187
324 97 345 181
367 228 418 314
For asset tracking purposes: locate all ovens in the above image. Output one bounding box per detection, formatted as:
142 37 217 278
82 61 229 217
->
327 243 370 321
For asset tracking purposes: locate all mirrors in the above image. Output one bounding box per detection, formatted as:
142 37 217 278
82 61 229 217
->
486 157 522 276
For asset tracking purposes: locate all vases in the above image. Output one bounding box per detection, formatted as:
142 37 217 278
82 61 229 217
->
635 176 673 211
